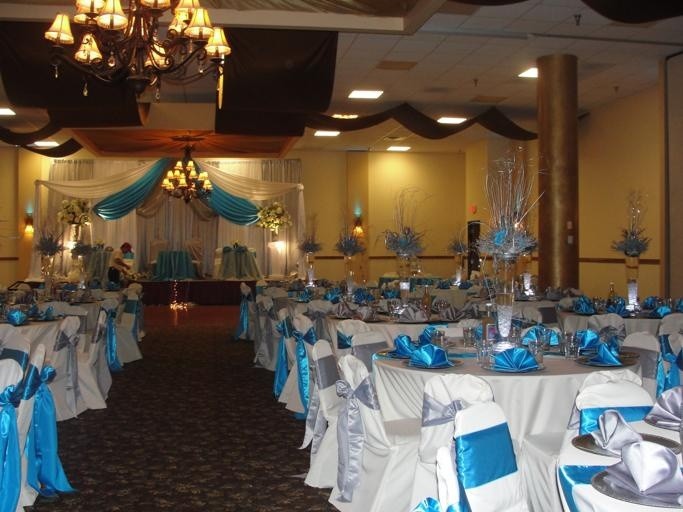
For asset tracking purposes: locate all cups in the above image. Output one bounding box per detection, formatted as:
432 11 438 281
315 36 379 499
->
428 324 578 369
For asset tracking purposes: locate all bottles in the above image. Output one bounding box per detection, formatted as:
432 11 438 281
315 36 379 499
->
422 284 432 316
606 281 616 304
480 303 495 346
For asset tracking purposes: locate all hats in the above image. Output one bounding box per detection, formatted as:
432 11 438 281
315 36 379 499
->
125 243 131 253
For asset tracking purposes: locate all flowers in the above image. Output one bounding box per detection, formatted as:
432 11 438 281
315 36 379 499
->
258 201 294 232
56 199 94 241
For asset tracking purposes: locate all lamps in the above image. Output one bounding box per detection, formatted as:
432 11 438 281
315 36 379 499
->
160 145 214 203
44 1 232 110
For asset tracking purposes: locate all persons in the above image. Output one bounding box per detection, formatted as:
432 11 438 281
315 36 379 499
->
108 242 133 283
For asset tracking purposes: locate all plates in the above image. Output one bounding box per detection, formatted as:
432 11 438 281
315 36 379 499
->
574 348 638 368
590 470 683 509
375 348 463 371
570 431 682 459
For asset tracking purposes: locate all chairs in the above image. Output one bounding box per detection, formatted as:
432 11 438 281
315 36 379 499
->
1 282 145 511
